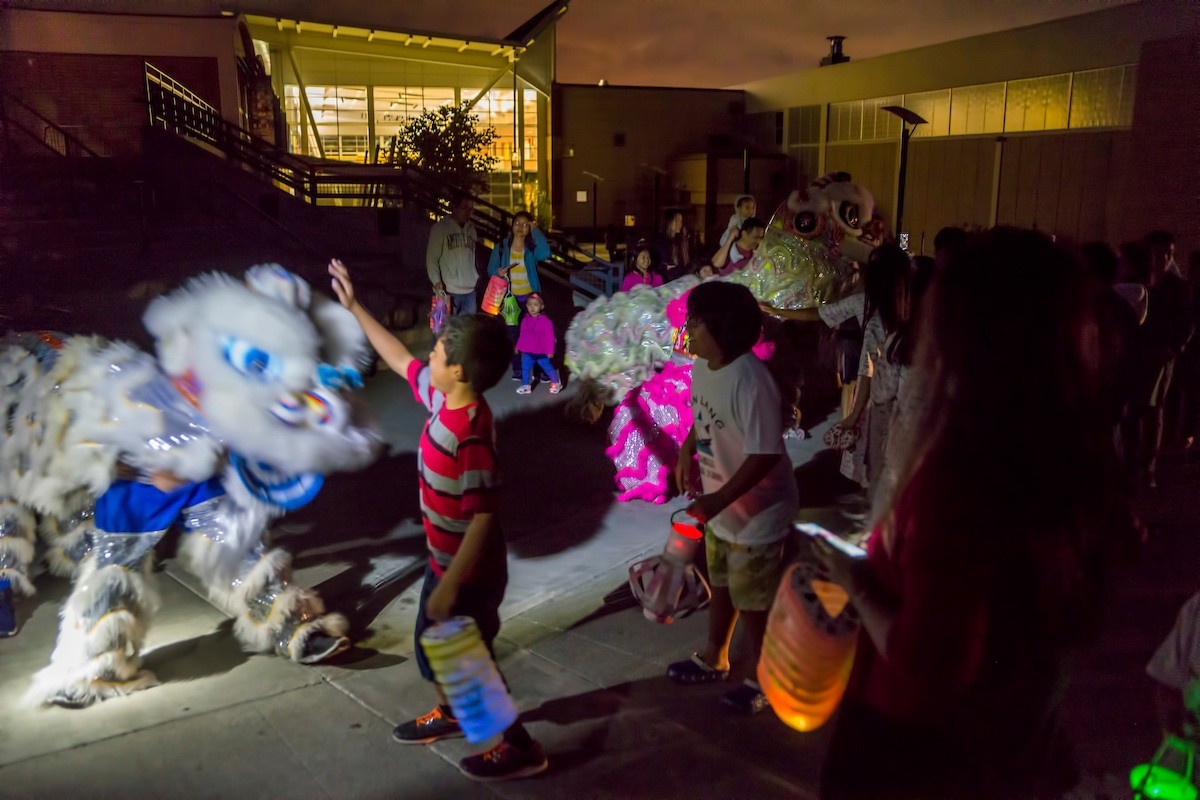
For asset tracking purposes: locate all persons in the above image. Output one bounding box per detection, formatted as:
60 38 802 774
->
820 224 1200 800
619 194 766 292
667 279 802 712
425 187 564 395
324 256 550 784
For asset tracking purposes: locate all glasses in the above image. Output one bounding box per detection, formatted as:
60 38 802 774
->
685 313 703 328
458 204 473 212
514 220 530 229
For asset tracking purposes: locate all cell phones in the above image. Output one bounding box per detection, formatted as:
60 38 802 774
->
793 520 867 560
899 232 909 250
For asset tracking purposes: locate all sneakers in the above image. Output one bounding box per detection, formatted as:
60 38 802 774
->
391 704 466 745
458 735 549 782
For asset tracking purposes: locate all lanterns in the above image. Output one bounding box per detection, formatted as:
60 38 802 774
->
481 275 508 315
757 561 858 732
421 618 519 743
430 295 450 330
500 291 521 326
1129 736 1199 800
628 508 711 624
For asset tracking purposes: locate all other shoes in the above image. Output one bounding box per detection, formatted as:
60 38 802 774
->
512 372 523 381
540 373 551 383
549 378 564 394
839 496 870 519
516 383 532 394
1145 472 1156 490
299 633 341 663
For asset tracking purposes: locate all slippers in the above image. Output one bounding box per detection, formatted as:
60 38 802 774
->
723 676 770 713
665 653 730 682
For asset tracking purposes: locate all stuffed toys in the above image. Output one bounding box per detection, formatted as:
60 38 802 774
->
0 263 390 709
564 170 886 428
